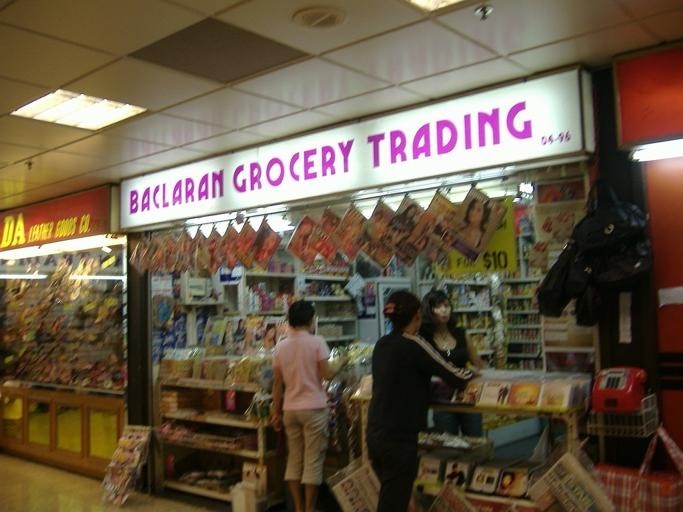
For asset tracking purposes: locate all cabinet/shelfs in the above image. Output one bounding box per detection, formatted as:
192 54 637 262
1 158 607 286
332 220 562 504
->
153 373 286 506
180 256 359 351
348 389 589 511
418 277 547 372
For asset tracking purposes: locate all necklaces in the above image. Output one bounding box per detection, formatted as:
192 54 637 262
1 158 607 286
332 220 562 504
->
439 330 450 356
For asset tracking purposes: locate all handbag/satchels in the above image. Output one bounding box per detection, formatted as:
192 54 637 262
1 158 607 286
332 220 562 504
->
570 178 652 282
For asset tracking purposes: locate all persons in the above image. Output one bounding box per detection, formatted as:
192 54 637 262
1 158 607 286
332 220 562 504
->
497 384 508 404
447 463 464 486
271 300 351 511
366 290 480 512
221 318 277 355
418 290 483 437
499 471 516 496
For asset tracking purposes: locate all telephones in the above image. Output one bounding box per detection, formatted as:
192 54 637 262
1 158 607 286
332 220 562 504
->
592 364 647 416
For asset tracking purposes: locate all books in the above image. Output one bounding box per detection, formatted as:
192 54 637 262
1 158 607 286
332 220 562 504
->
129 218 281 276
286 188 508 276
